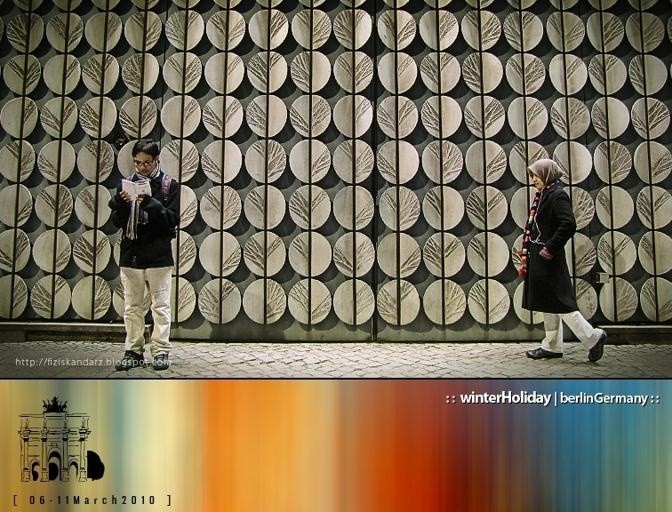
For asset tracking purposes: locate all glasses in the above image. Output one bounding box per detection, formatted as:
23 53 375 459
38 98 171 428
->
133 157 155 165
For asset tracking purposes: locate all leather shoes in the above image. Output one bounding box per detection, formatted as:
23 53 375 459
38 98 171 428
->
526 347 563 359
588 329 607 362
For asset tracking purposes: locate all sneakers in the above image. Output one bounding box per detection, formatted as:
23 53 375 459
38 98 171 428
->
153 354 169 370
114 349 145 371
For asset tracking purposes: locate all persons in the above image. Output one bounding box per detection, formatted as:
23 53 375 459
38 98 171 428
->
108 138 180 371
517 158 607 363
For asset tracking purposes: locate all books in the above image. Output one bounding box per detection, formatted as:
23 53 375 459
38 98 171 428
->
121 179 152 202
